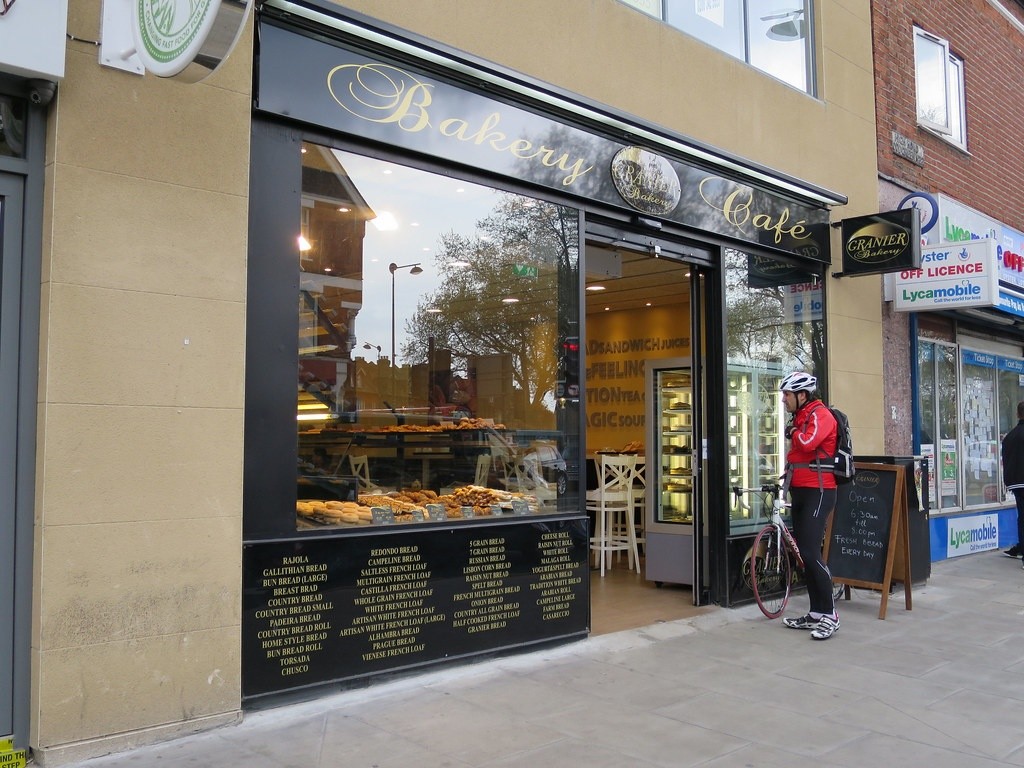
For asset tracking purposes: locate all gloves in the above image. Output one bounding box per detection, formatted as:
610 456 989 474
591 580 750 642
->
784 425 798 439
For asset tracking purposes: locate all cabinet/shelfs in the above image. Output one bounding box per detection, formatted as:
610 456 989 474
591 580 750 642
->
644 356 792 589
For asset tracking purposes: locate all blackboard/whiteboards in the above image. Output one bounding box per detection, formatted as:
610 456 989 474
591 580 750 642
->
822 461 912 591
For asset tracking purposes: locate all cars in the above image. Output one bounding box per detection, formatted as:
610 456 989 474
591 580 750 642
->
964 440 1005 489
518 445 568 495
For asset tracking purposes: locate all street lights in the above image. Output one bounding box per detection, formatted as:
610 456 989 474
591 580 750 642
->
388 262 423 408
363 341 381 360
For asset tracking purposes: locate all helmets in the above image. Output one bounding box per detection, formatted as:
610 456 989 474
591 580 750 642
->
779 372 817 394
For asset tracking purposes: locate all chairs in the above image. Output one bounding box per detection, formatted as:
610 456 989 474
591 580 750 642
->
501 452 646 578
348 454 377 489
474 454 491 488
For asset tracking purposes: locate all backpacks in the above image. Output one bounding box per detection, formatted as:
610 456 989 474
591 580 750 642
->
809 408 855 485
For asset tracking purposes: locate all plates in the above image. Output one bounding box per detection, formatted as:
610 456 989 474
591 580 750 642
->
670 405 690 409
672 426 692 431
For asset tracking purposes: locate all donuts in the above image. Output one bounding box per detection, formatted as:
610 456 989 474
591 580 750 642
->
296 499 373 522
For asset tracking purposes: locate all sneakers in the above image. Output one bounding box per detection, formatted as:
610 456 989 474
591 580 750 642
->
810 612 840 640
782 613 820 631
1003 546 1024 559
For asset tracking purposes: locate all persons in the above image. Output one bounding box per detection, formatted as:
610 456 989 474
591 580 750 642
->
779 372 840 640
1002 402 1024 570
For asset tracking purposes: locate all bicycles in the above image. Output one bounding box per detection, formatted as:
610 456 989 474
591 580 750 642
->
733 484 845 619
